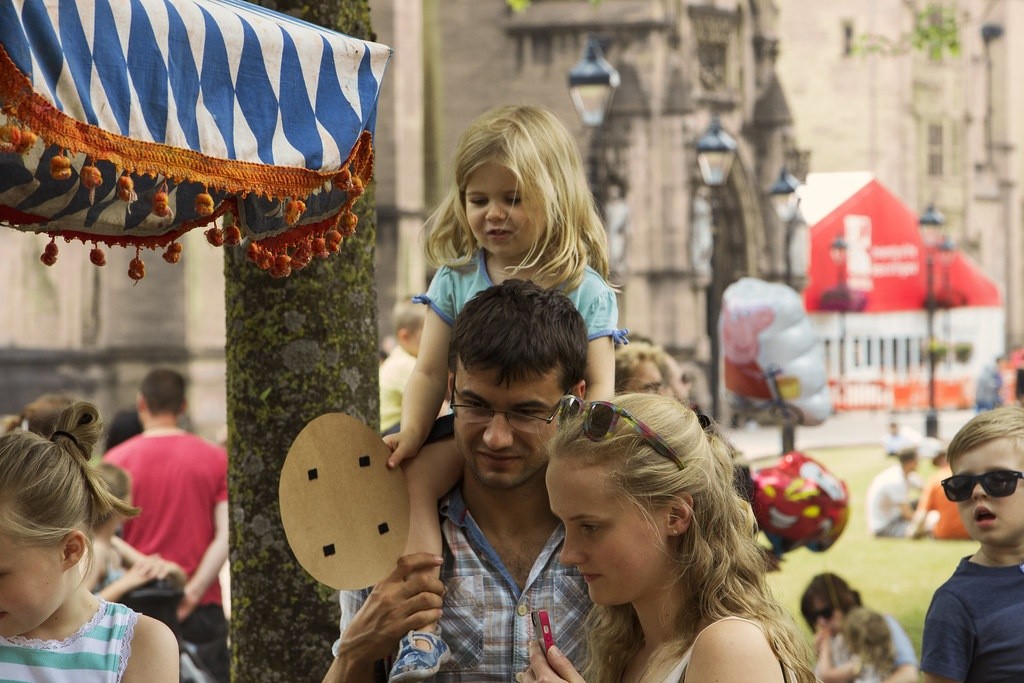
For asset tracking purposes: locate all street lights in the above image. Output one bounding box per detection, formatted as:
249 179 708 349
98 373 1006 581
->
566 36 621 187
767 163 805 457
694 113 740 427
917 198 948 441
829 231 851 409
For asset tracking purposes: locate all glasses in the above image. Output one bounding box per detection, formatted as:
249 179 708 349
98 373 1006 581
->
450 372 573 434
556 396 688 472
941 470 1024 502
810 605 834 624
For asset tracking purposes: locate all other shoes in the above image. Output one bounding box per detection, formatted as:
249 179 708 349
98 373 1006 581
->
388 624 450 683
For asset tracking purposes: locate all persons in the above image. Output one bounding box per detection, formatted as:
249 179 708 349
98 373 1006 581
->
0 369 231 683
383 104 627 683
322 280 588 683
377 296 1024 683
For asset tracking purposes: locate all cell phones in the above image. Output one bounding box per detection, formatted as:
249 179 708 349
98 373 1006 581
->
531 610 554 656
